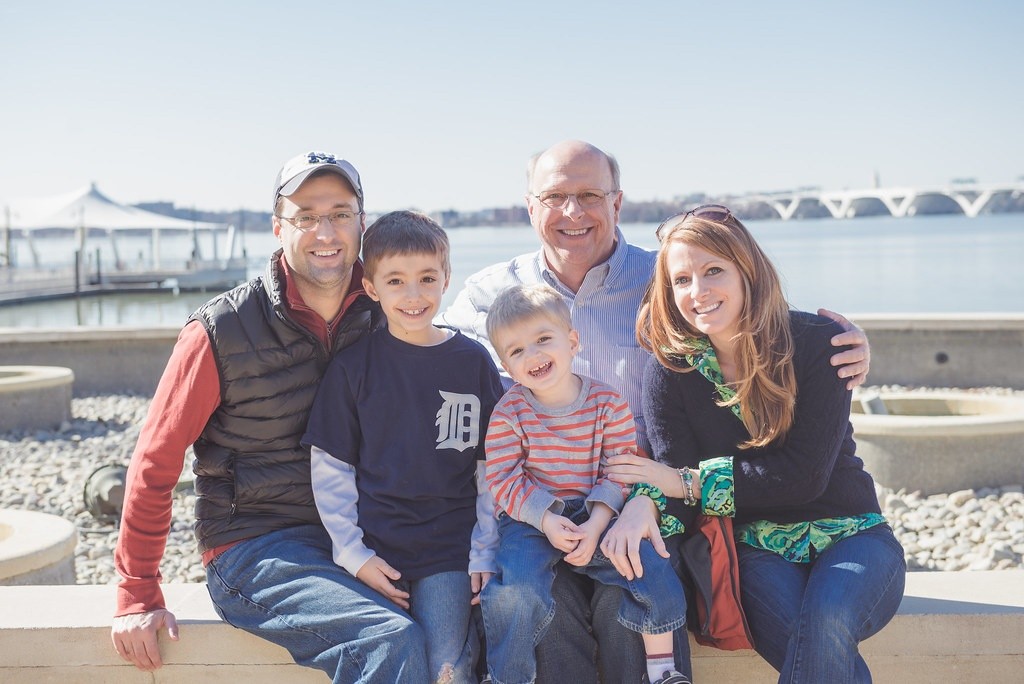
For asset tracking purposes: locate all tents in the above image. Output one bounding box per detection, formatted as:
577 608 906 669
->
1 183 237 272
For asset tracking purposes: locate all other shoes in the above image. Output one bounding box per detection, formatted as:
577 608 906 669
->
642 669 691 684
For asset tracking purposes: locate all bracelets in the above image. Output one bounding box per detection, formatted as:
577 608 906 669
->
677 466 697 508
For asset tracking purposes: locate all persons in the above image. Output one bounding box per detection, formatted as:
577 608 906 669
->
301 210 507 684
435 141 874 684
109 153 428 683
603 206 907 684
484 284 688 684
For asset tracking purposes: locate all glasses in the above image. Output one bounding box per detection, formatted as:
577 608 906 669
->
530 189 618 210
275 210 364 230
656 203 731 243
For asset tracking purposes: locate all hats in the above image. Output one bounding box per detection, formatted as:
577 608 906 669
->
273 152 361 207
